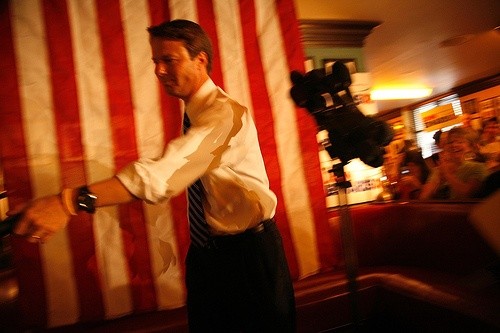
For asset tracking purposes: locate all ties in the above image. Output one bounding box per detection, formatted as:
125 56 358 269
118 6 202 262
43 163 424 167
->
182 112 207 251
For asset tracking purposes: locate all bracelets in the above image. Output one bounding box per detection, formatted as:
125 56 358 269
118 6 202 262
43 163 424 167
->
61 188 77 216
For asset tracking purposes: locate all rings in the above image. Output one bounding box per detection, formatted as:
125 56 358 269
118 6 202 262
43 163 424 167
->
31 235 41 239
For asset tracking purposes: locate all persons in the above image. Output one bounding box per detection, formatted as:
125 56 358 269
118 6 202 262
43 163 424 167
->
5 19 297 333
389 115 500 264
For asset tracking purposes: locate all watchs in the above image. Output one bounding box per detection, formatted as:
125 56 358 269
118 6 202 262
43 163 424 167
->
75 185 97 213
